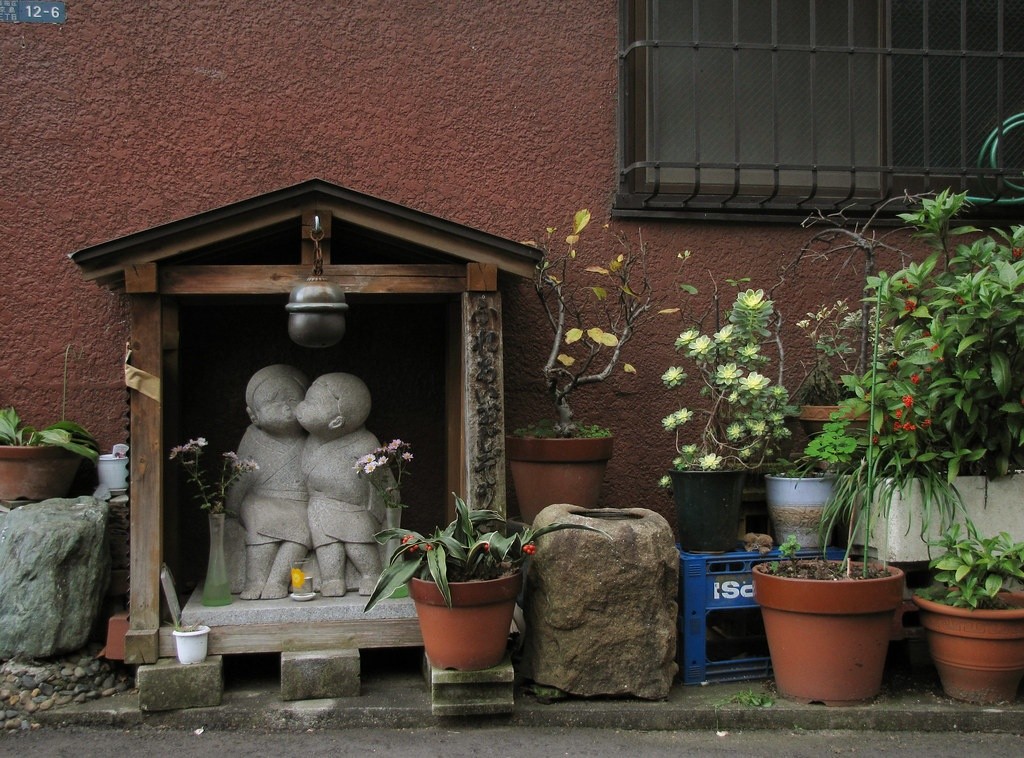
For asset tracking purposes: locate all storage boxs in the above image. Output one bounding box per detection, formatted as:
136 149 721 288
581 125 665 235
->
676 542 850 687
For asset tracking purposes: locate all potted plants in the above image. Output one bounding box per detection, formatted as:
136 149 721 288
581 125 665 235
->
787 183 1024 562
752 538 904 708
913 518 1024 707
657 277 801 548
361 491 614 672
504 210 686 525
163 616 211 664
0 406 100 501
763 452 844 551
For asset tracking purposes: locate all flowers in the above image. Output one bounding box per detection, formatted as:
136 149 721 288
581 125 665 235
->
353 438 415 509
169 438 259 513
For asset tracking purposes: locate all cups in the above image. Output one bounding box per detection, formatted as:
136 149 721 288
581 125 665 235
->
289 558 315 596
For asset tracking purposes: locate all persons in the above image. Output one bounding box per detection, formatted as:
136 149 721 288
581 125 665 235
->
223 365 402 600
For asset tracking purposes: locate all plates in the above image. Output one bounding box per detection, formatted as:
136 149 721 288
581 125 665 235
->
290 593 316 601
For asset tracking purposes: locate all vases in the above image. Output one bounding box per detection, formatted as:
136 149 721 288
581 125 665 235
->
203 513 233 606
385 506 409 599
